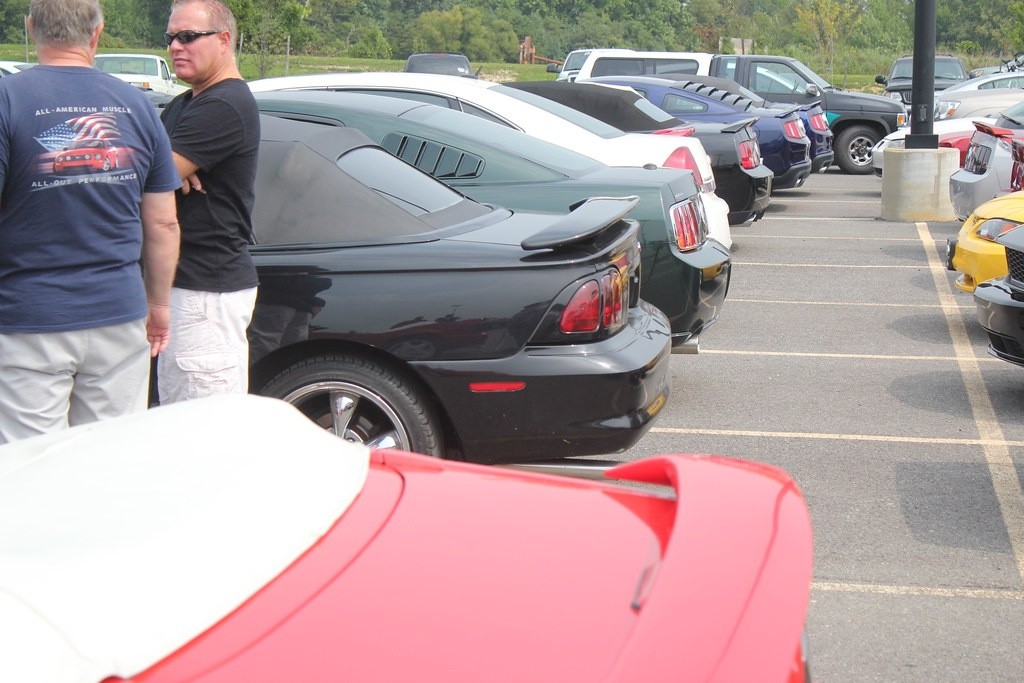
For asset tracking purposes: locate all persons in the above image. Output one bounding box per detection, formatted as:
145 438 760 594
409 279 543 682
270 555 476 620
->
158 1 260 406
0 1 179 444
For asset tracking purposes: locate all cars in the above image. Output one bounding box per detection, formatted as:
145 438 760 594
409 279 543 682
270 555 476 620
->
871 107 1008 178
602 77 812 191
948 120 1024 221
934 72 1024 96
547 49 593 81
404 52 482 79
593 75 772 233
249 95 733 355
934 87 1024 123
246 68 735 249
95 53 176 94
54 137 118 174
949 188 1024 308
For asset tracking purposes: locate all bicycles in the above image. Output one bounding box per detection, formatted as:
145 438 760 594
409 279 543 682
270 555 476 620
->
992 52 1024 74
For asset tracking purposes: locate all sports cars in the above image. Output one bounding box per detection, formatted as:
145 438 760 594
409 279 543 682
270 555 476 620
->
139 100 676 469
504 81 772 232
663 72 835 176
0 390 814 682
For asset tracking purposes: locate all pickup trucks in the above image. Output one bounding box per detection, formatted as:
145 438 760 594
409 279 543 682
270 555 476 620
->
710 52 906 178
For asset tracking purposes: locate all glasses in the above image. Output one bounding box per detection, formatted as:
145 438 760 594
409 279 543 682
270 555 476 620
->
163 29 221 45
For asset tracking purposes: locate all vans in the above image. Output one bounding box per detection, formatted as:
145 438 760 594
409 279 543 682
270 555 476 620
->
574 50 807 95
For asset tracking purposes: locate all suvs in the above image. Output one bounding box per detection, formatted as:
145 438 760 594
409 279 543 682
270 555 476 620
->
876 54 967 112
